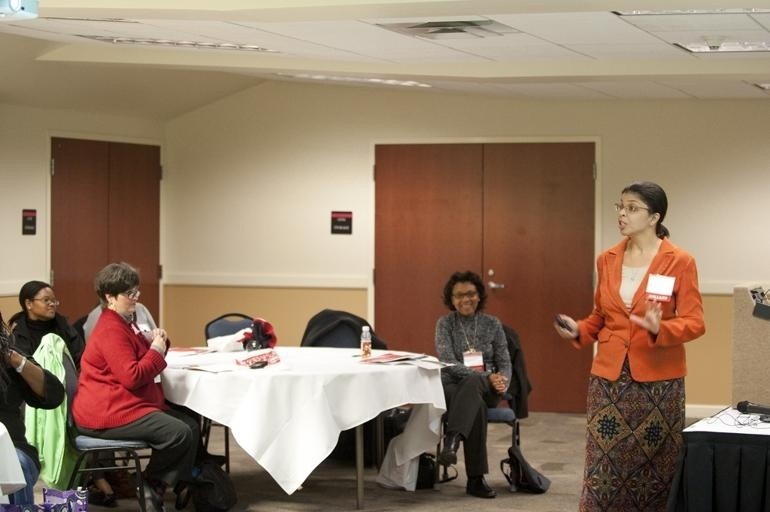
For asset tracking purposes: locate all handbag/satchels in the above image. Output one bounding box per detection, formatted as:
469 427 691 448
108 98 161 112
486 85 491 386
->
416 452 458 490
192 463 237 511
499 445 552 494
0 488 89 512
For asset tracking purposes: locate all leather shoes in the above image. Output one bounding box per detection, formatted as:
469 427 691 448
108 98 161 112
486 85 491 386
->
438 431 461 466
466 475 497 499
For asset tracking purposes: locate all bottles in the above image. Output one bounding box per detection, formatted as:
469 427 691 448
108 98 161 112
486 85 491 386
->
361 326 372 358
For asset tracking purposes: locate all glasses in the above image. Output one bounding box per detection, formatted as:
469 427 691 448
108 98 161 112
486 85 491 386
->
614 202 650 214
32 297 60 306
451 291 477 298
125 291 142 299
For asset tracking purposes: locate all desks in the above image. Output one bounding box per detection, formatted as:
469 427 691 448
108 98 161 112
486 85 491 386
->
666 406 770 512
159 348 448 510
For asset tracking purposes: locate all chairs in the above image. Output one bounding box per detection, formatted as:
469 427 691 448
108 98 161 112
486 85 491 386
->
303 310 373 348
45 333 152 511
436 325 520 493
205 313 254 346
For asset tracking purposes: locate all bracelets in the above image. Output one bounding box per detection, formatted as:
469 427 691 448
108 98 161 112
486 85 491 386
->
16 356 27 374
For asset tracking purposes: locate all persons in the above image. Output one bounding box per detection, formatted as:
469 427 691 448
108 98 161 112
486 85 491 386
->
81 303 227 496
0 311 65 512
6 280 118 509
434 269 512 498
552 180 707 511
70 260 202 511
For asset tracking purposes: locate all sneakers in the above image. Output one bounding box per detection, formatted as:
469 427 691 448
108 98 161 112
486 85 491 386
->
129 472 146 491
137 479 166 512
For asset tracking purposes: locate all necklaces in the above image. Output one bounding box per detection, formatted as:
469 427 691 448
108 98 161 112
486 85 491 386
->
628 246 650 282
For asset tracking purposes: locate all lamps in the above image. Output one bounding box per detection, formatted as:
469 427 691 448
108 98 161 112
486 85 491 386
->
0 1 39 22
